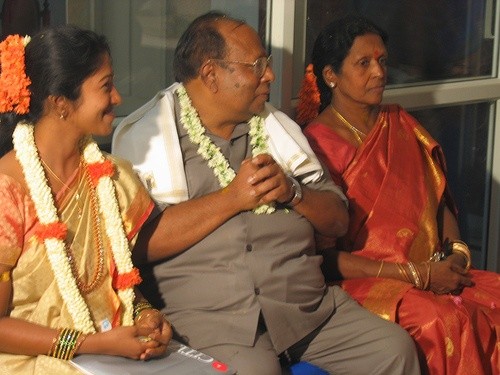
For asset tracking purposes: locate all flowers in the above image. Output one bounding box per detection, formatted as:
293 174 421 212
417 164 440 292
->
0 33 31 116
176 82 278 215
11 120 145 336
295 63 322 127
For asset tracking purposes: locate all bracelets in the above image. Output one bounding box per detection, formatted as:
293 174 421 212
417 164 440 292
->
48 329 87 360
395 260 431 291
446 240 471 273
376 260 383 277
132 300 154 316
284 176 303 210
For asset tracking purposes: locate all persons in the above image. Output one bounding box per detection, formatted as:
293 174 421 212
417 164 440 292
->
111 11 422 375
300 15 500 375
0 25 239 375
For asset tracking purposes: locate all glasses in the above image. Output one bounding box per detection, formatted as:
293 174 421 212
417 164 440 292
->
208 55 273 76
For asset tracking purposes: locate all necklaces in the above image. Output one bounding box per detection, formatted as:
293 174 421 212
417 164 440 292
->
328 103 368 147
13 118 142 335
176 81 278 215
